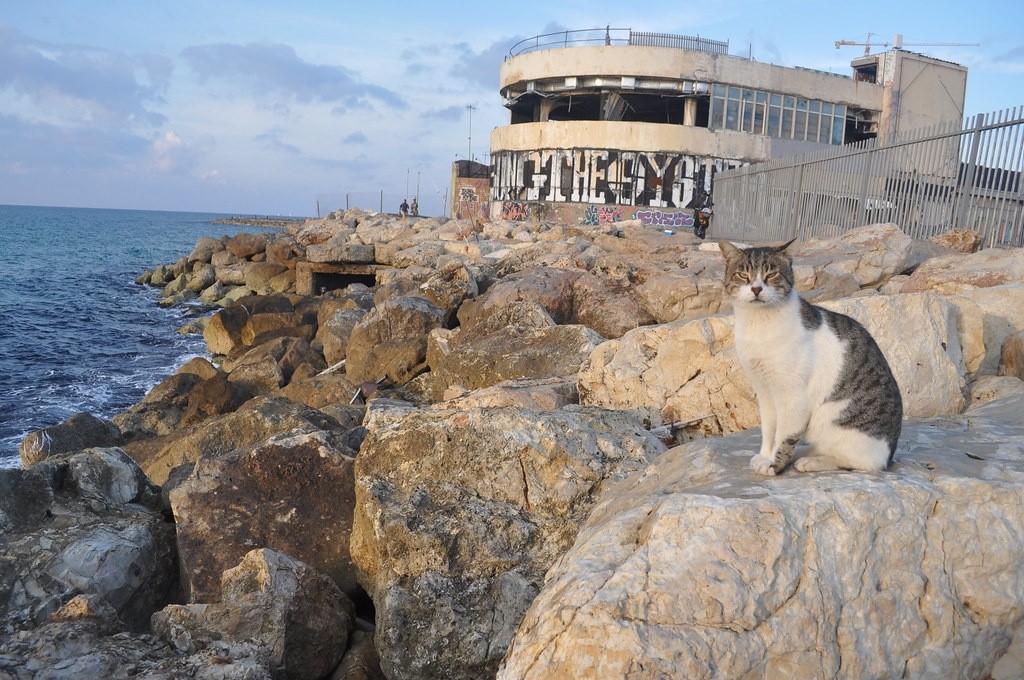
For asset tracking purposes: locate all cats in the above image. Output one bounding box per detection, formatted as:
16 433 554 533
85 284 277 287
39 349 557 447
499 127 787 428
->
718 237 902 477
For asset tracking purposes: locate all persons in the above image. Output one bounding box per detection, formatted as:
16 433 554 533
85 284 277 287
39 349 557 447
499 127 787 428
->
411 199 418 216
400 199 409 217
693 190 712 239
